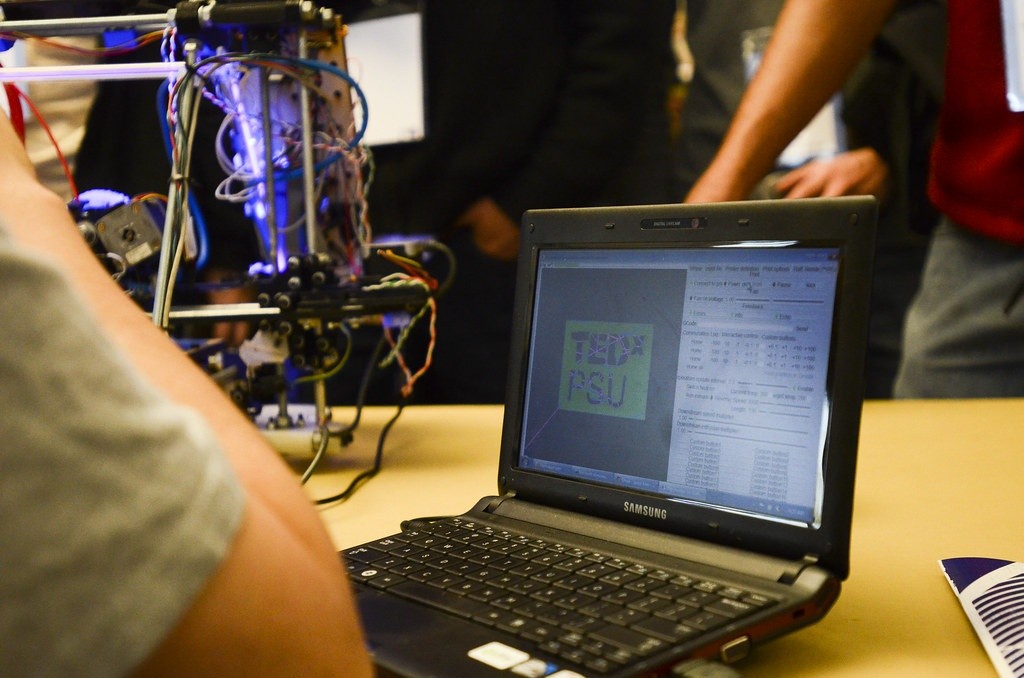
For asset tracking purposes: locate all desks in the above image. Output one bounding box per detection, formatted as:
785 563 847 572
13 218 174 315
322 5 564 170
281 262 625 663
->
244 398 1024 678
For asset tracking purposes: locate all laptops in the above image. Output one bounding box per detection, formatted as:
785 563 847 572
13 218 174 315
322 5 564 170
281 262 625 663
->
337 193 879 678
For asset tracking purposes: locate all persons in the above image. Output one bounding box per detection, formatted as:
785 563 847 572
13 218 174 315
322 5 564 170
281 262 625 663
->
681 0 1024 399
281 0 677 404
0 0 371 676
